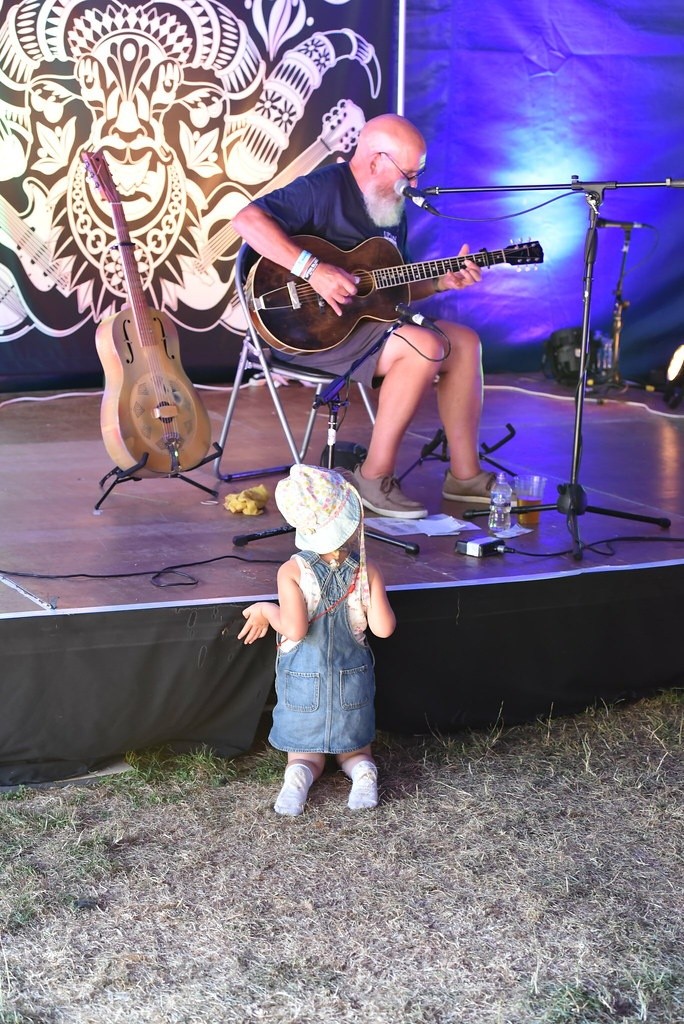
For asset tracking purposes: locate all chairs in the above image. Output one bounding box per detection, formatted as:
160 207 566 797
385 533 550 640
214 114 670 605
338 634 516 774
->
214 241 375 481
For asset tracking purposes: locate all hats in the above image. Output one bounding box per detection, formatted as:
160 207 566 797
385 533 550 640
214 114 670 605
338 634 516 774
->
275 463 360 555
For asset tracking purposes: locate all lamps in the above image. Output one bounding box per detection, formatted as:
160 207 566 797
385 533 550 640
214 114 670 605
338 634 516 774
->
663 343 684 408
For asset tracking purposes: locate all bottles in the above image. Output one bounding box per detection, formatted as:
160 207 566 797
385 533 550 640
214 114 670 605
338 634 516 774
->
489 471 513 531
594 330 614 370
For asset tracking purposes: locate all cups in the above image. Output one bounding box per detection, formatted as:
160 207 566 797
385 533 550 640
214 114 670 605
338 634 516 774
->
514 475 548 526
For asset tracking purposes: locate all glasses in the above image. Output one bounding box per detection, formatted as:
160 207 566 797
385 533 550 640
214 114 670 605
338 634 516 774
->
376 152 427 182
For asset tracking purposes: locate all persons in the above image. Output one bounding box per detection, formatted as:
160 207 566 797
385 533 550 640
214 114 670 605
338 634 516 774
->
234 464 396 817
231 113 523 519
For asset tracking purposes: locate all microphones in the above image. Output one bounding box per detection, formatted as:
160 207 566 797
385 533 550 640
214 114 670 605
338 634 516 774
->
395 179 439 217
595 217 641 229
394 301 443 333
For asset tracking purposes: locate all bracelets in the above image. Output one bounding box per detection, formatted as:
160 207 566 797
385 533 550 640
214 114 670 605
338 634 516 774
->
302 256 319 282
291 250 312 277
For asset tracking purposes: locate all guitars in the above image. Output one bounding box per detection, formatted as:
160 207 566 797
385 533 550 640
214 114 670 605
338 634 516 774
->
78 143 215 480
242 235 546 357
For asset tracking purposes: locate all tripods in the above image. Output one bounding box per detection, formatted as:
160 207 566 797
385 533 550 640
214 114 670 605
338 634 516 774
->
392 180 684 563
231 315 420 553
95 242 222 509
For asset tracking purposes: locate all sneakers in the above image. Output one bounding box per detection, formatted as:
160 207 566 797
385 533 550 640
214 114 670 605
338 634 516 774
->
355 465 428 520
442 464 519 507
